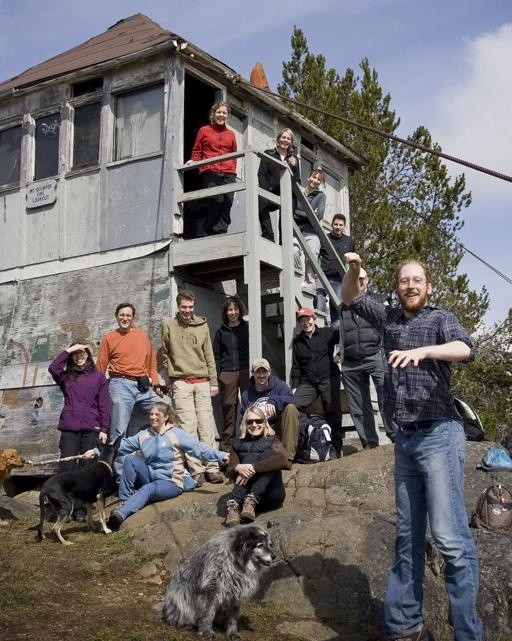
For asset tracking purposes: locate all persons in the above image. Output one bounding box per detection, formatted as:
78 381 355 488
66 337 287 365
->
342 248 488 641
93 303 172 466
213 295 249 446
82 403 231 529
48 340 110 522
160 293 226 487
184 100 238 235
259 126 302 243
292 165 327 288
234 359 303 469
338 268 396 450
289 309 341 460
316 214 355 326
224 405 291 527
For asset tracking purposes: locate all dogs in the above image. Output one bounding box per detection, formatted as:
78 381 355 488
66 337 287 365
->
24 431 125 546
151 524 278 641
0 448 26 526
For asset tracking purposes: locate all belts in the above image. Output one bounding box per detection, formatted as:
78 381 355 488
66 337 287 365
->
112 373 137 382
396 420 434 431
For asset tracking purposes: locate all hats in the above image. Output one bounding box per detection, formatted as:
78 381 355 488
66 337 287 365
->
296 306 315 321
251 358 271 372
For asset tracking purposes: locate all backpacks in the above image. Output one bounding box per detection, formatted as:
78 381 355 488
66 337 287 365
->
470 482 512 531
454 396 485 442
295 414 334 463
479 444 511 472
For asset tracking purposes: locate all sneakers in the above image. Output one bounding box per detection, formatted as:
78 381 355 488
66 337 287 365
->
226 499 241 527
239 494 257 522
192 472 205 487
109 512 124 529
50 507 88 524
205 471 223 485
